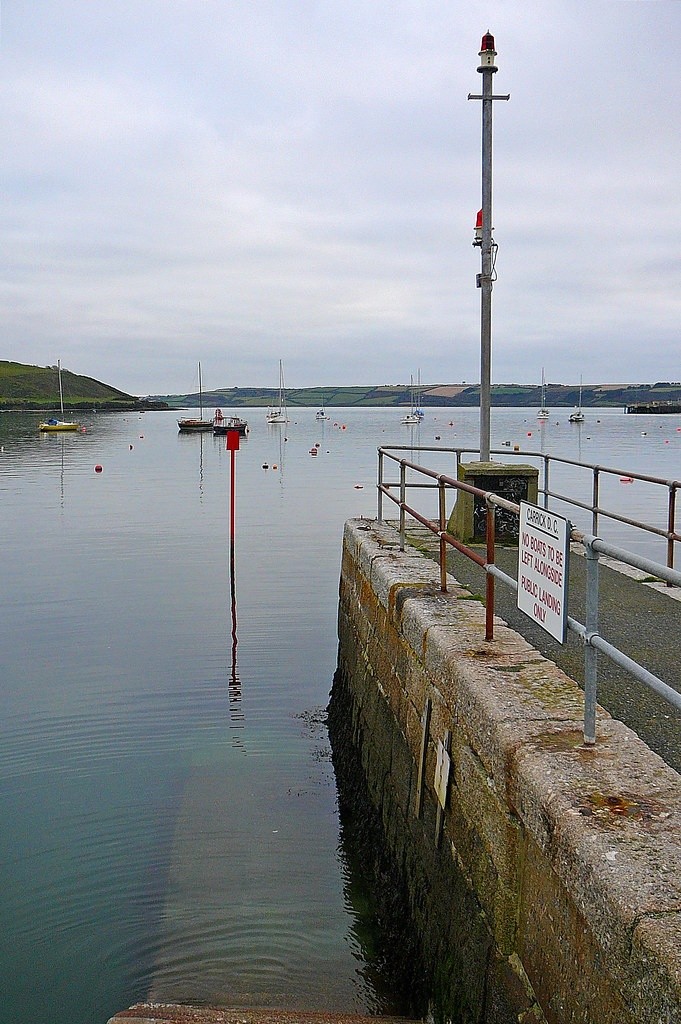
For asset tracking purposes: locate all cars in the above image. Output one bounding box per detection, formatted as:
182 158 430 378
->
666 401 673 406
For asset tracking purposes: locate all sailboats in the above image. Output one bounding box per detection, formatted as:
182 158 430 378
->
266 359 290 423
568 374 585 422
537 367 550 419
402 375 420 424
178 361 213 431
413 369 424 418
39 360 78 430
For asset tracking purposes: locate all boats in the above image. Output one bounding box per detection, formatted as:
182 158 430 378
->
213 409 249 438
316 409 327 420
